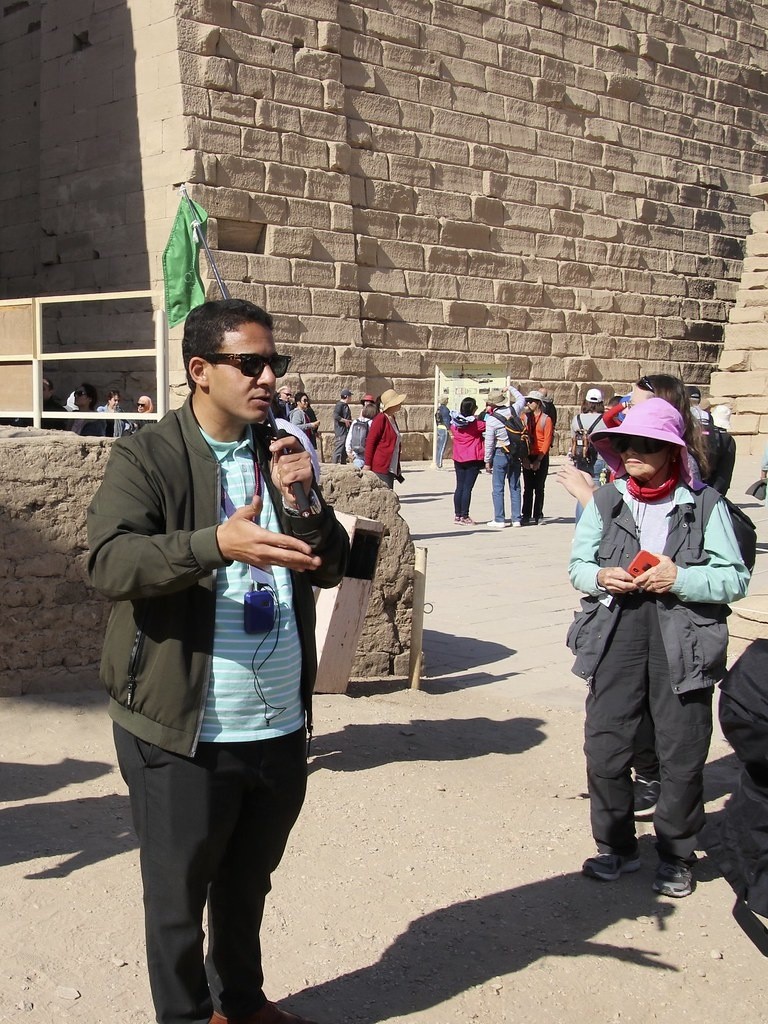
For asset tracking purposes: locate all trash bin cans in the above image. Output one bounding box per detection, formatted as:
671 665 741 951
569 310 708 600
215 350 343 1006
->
313 512 385 696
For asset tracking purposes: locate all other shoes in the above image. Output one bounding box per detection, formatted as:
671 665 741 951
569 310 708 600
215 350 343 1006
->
210 998 303 1024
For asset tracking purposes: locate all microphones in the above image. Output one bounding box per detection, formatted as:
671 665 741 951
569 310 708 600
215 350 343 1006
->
277 428 310 517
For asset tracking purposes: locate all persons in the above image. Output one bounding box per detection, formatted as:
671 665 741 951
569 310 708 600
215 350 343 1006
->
477 386 557 528
435 394 452 470
274 386 294 422
333 389 354 465
554 373 711 816
450 397 487 526
88 298 353 1024
288 393 320 450
567 396 751 897
716 635 768 916
568 387 736 531
345 389 407 489
0 377 156 437
759 447 768 510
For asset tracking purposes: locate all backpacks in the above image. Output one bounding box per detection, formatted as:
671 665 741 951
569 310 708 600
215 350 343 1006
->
491 408 533 458
351 418 372 453
573 412 604 465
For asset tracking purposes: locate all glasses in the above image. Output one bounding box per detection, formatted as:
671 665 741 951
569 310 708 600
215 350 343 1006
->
641 376 655 394
198 349 291 379
616 433 668 454
137 403 147 408
74 391 88 397
300 399 310 403
526 398 537 404
281 392 292 396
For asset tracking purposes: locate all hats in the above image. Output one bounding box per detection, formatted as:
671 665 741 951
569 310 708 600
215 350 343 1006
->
711 405 733 429
587 397 709 491
524 391 546 407
361 395 375 405
341 390 354 398
684 385 701 399
585 389 604 403
488 393 509 406
381 389 408 412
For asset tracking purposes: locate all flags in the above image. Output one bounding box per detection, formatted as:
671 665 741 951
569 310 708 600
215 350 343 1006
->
162 195 208 330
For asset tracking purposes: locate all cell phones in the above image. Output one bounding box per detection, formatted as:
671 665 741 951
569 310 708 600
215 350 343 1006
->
395 474 405 483
626 550 660 578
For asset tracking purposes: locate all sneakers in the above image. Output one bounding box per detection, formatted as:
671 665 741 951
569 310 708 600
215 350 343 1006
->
631 773 662 816
582 846 641 879
487 517 547 528
454 516 476 525
653 862 692 896
